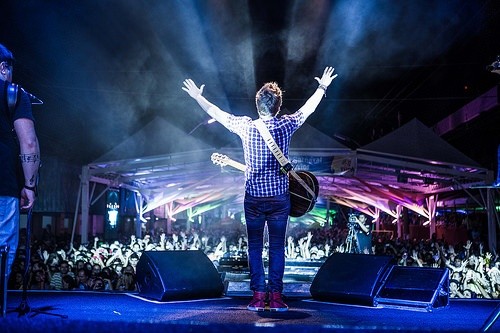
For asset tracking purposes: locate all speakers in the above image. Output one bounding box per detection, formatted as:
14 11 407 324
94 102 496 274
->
309 251 398 307
373 265 449 310
133 249 224 302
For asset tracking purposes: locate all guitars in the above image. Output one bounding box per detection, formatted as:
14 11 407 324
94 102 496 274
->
210 152 319 218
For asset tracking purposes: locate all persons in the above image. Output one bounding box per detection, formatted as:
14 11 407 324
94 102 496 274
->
7 213 500 300
0 43 42 318
182 66 338 312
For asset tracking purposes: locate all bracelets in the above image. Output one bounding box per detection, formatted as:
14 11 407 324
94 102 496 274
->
24 185 36 192
318 85 327 90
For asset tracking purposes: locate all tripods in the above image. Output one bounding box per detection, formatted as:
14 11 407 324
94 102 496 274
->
6 207 68 318
345 225 361 254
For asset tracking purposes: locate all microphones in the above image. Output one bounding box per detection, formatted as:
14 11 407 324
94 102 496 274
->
20 87 39 100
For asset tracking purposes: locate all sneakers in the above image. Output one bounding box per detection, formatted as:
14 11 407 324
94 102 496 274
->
270 292 288 311
247 291 266 311
